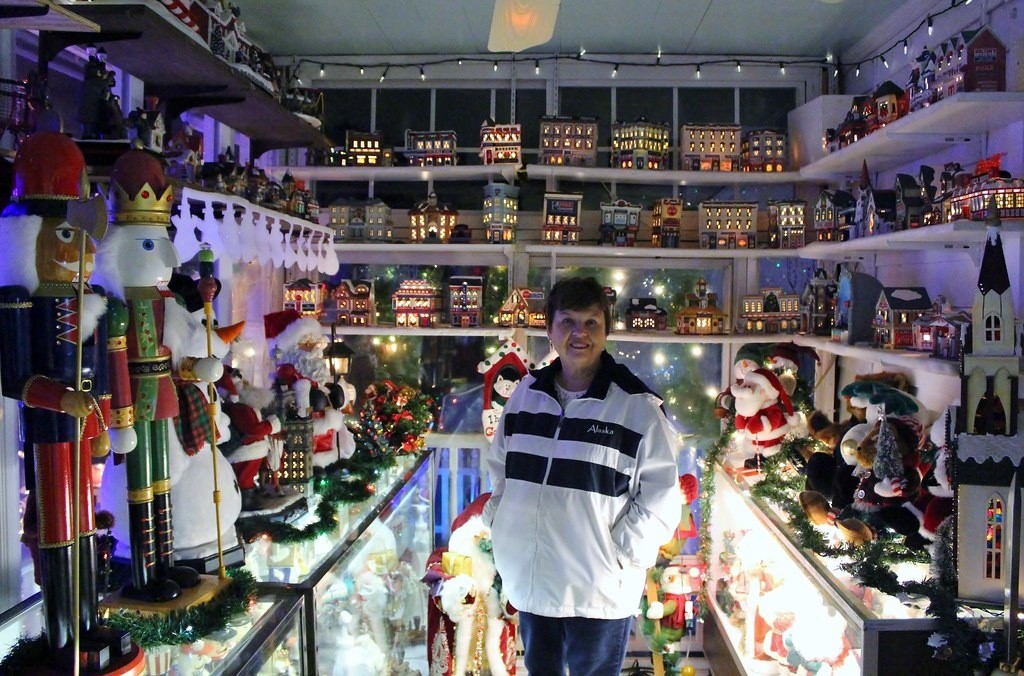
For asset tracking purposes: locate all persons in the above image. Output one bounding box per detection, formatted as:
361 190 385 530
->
425 491 519 676
0 133 245 676
731 369 793 469
483 276 682 676
264 309 372 513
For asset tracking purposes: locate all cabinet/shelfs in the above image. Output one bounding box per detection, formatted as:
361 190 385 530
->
0 0 1024 676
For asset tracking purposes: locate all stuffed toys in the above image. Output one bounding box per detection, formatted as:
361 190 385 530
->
715 343 952 545
